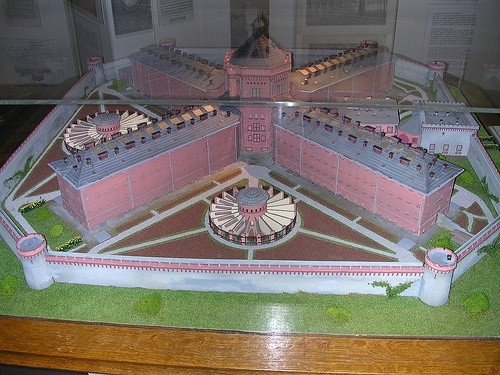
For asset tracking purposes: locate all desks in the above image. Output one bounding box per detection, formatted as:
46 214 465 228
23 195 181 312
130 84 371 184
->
0 47 500 375
